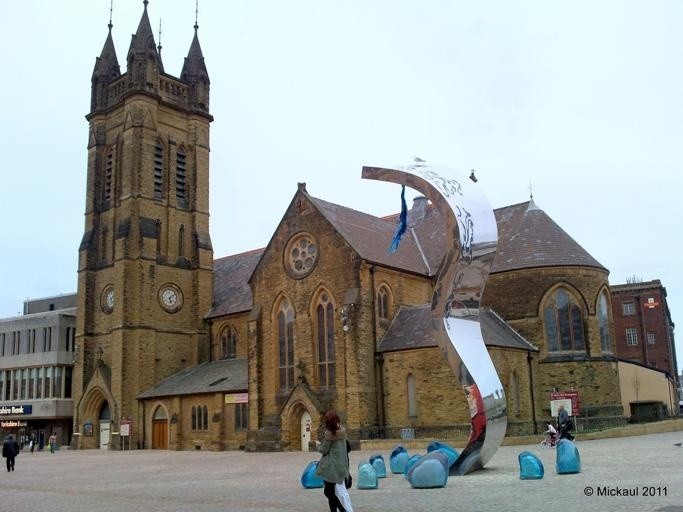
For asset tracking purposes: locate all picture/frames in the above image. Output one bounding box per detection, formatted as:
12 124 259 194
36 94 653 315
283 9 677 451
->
84 424 93 437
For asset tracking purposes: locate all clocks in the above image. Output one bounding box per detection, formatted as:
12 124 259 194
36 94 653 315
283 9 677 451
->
159 286 181 310
103 287 114 311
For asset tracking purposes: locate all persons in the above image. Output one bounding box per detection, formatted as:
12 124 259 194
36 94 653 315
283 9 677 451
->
50 432 56 450
48 433 55 453
28 438 34 456
0 434 19 472
546 420 557 447
312 409 351 511
557 403 576 443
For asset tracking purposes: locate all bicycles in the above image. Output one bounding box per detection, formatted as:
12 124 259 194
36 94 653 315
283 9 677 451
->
540 432 560 448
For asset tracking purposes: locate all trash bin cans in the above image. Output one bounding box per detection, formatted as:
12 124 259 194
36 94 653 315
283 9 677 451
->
73 434 82 450
629 401 663 422
309 442 313 452
680 405 683 414
